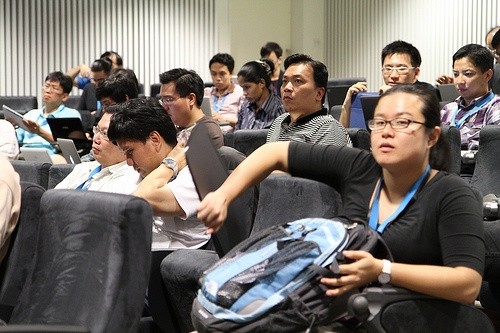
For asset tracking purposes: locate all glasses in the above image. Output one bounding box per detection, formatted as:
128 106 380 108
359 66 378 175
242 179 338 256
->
367 117 428 131
160 95 186 105
41 85 60 91
119 139 142 157
92 126 108 139
382 66 415 74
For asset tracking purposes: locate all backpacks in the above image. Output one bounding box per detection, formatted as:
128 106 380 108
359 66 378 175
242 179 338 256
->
190 214 394 333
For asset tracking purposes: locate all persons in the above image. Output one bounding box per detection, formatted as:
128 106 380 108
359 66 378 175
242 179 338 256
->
79 59 112 126
340 40 422 129
196 83 488 333
158 66 223 153
108 97 214 333
440 44 500 175
5 71 81 156
64 52 124 91
53 105 140 196
267 53 354 176
200 43 287 134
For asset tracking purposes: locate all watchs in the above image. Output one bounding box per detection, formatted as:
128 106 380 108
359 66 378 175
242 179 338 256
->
377 259 392 285
161 156 178 180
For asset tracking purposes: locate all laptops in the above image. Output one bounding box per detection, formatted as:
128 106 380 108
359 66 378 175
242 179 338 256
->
47 118 93 146
57 138 81 164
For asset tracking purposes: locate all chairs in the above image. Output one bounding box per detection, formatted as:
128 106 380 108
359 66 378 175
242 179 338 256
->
1 78 500 333
